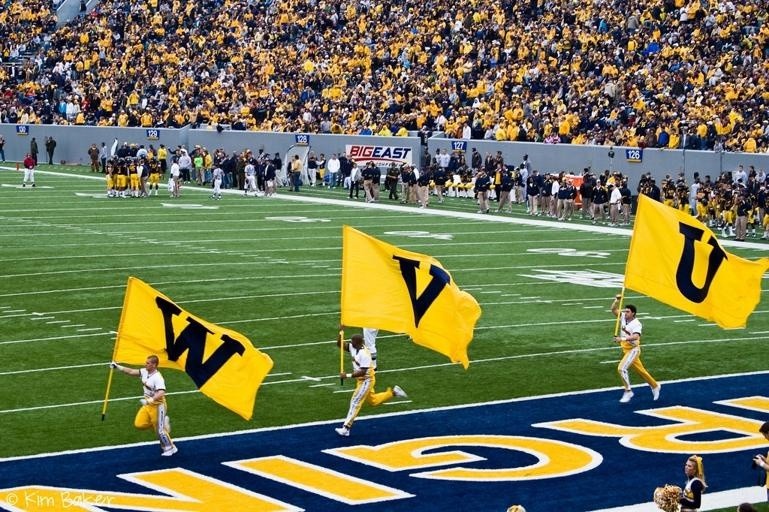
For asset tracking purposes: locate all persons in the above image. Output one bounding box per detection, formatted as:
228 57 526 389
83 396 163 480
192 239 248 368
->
108 355 179 457
676 455 708 512
0 0 769 242
611 295 661 407
363 328 379 371
737 503 757 512
334 325 407 436
753 420 769 502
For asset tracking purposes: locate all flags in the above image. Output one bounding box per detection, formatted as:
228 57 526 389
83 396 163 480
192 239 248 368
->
622 192 767 330
115 274 276 421
337 224 481 369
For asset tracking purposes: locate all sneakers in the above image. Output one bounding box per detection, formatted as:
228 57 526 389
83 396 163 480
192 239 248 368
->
335 428 350 437
161 416 177 457
652 384 661 401
619 391 634 403
393 385 407 397
